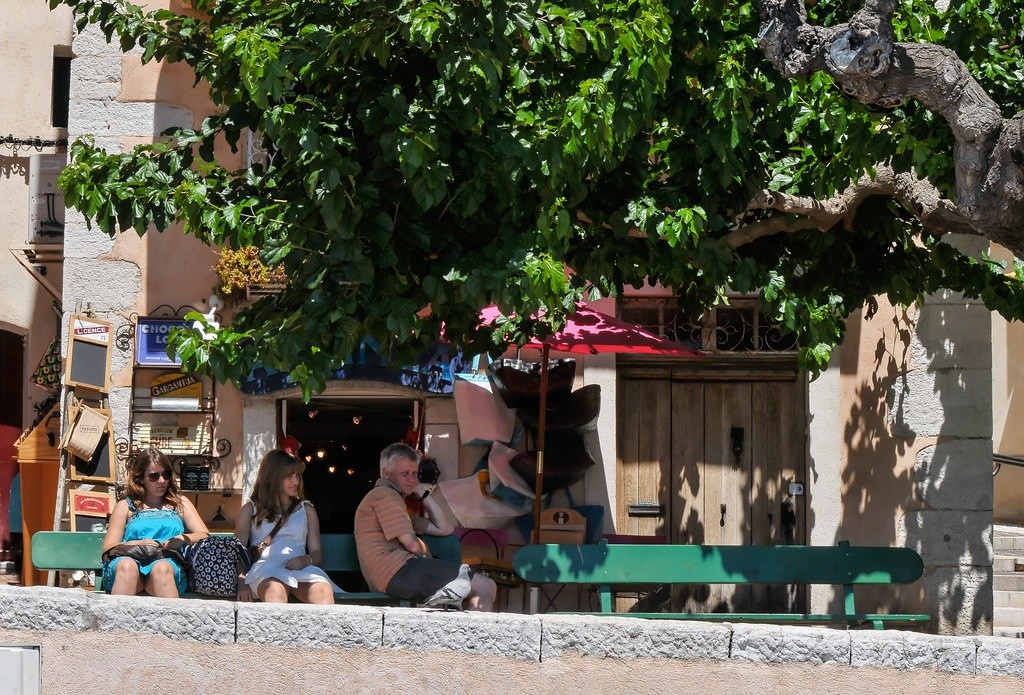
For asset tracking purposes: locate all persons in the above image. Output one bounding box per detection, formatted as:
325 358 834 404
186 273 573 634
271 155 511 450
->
355 444 497 612
102 448 210 597
234 450 345 604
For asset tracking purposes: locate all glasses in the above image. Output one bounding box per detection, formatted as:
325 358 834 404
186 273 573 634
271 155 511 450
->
141 470 173 481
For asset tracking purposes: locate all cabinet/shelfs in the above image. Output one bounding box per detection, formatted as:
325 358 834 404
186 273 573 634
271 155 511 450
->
117 306 232 509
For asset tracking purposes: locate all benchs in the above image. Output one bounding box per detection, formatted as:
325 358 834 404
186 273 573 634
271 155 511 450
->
513 537 932 630
31 531 462 607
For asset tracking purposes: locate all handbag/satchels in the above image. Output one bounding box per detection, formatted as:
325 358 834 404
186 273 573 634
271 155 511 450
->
30 317 62 393
247 534 264 565
439 352 605 544
185 535 252 597
101 539 191 582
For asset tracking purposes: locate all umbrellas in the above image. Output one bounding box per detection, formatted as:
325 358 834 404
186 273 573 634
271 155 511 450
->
442 298 706 544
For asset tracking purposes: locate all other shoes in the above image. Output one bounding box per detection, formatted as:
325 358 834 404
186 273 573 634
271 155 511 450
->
627 585 672 612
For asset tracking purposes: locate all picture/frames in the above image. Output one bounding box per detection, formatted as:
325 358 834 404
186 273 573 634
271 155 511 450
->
136 315 196 365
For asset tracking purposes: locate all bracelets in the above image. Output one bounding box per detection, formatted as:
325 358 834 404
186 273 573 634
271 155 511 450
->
307 555 312 564
419 490 429 501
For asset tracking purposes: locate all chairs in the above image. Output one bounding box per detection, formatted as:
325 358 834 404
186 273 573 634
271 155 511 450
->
603 534 667 612
454 527 510 612
538 507 588 612
